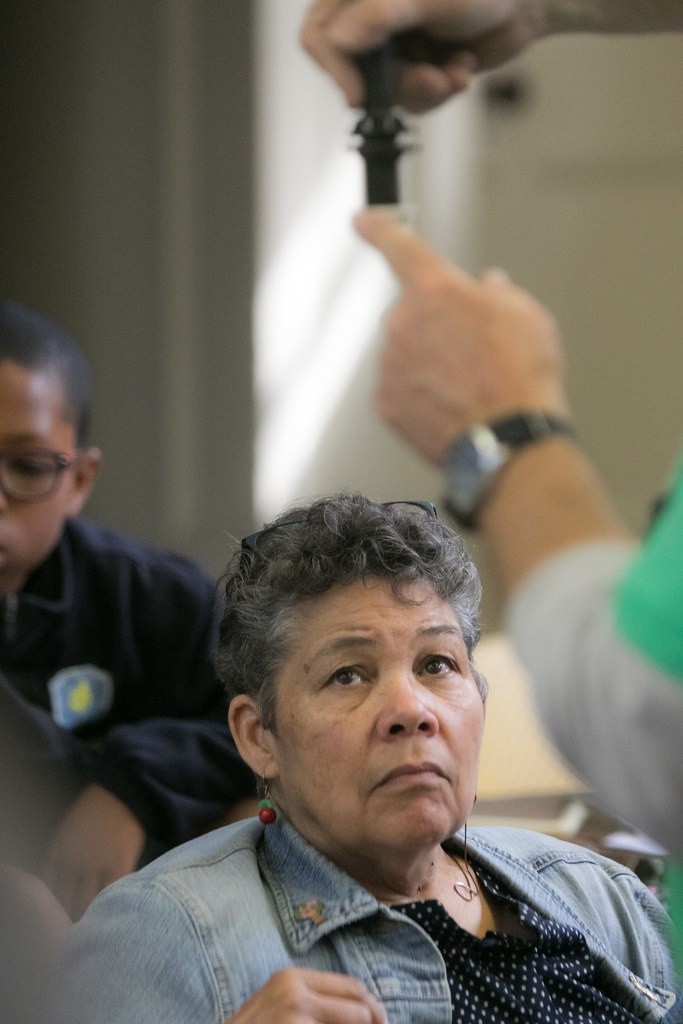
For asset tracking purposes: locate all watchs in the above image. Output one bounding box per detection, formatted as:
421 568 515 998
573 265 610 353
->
439 410 575 532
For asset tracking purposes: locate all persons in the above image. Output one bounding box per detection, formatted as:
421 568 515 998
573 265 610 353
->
61 497 683 1024
0 305 265 923
300 0 683 858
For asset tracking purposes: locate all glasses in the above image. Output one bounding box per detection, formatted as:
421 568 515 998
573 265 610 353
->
238 500 439 575
0 447 82 501
